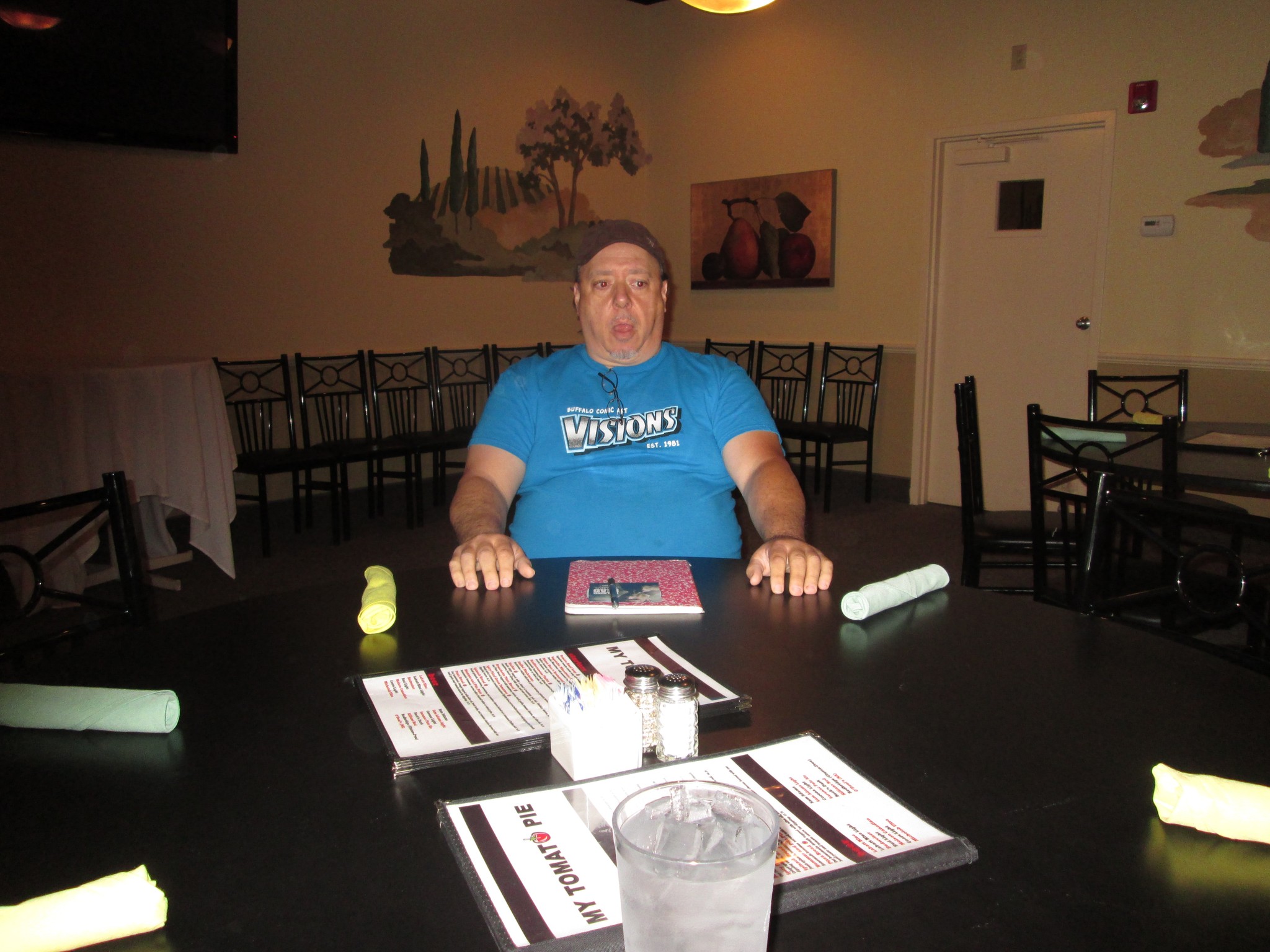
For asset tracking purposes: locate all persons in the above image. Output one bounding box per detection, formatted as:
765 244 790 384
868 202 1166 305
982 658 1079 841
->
448 220 833 597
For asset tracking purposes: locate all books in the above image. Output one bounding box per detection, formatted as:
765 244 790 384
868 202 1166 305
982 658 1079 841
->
564 560 706 615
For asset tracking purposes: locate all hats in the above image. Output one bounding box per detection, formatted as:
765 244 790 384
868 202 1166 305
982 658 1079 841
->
569 220 662 267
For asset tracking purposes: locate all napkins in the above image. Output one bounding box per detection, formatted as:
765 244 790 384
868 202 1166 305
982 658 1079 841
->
0 682 180 732
1041 422 1270 498
357 565 396 634
1153 764 1270 844
1133 411 1164 425
1043 427 1127 442
0 864 168 952
841 564 950 620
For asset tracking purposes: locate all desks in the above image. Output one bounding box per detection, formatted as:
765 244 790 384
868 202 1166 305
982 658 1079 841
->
0 556 1270 951
0 357 238 617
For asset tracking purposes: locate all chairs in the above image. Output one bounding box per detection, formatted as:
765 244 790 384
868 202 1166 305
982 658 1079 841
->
705 338 883 513
956 370 1270 681
212 341 543 557
1 471 148 671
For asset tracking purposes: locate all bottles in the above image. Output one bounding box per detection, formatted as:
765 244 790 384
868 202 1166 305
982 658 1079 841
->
621 663 662 753
655 673 698 764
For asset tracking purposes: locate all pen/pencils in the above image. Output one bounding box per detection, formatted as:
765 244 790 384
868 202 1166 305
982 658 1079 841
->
609 578 619 609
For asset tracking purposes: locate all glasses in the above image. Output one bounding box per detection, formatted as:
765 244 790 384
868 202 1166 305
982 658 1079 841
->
598 368 624 425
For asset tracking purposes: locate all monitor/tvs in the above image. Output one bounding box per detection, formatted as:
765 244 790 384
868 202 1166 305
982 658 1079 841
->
0 0 239 153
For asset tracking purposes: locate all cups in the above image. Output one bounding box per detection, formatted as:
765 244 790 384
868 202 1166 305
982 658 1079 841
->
613 780 780 952
